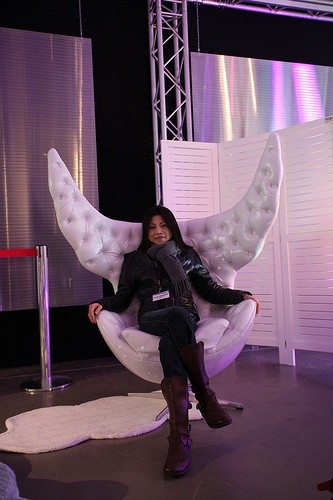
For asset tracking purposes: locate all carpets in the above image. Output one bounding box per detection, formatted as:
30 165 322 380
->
0 388 204 455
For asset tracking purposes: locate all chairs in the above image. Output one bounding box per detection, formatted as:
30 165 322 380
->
45 132 284 421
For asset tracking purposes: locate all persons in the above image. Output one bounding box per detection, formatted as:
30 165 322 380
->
88 205 260 478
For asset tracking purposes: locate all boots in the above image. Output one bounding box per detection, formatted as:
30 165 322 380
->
178 340 234 429
160 376 194 477
